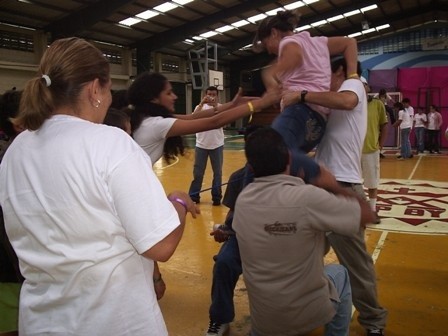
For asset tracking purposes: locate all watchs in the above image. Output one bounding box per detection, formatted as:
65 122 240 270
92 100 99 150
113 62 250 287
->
301 89 308 103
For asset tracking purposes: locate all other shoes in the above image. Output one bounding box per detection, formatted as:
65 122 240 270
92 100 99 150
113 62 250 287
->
373 211 380 224
213 223 235 235
365 322 385 336
212 200 220 206
191 196 200 203
206 320 229 336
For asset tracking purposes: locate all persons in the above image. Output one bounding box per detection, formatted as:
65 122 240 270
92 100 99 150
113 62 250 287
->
188 86 225 206
0 89 27 336
102 72 283 301
0 36 201 336
207 10 388 336
378 90 443 159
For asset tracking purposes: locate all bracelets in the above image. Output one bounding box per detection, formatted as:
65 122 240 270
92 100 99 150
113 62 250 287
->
153 273 162 285
347 73 358 78
168 196 188 214
213 105 219 115
247 101 260 123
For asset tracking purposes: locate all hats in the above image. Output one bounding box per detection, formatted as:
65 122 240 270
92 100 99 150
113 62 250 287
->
360 76 368 84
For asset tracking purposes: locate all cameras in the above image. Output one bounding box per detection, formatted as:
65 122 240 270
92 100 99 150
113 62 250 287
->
208 97 216 103
